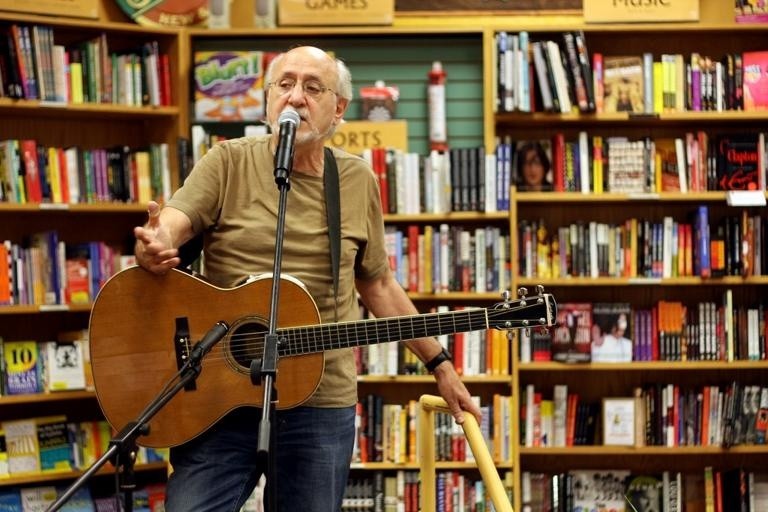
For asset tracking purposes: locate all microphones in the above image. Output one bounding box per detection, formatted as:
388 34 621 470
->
191 320 229 363
275 110 301 185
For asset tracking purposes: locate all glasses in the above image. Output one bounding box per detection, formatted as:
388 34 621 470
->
269 77 337 97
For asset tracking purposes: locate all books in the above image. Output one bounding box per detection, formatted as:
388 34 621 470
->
363 142 485 213
192 123 271 167
355 305 510 375
518 466 768 511
515 290 768 362
494 30 767 113
508 129 768 193
193 48 267 120
517 204 767 279
520 379 768 448
1 18 174 509
342 394 513 511
382 222 511 292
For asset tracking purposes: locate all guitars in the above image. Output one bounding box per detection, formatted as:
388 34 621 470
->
89 263 558 448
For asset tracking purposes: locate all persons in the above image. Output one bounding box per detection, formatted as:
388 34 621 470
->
134 44 483 511
